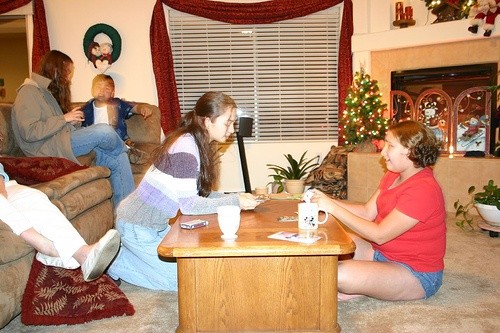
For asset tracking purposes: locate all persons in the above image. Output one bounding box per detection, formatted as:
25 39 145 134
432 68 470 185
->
0 162 120 281
89 43 113 76
468 0 500 36
312 121 447 301
11 50 147 207
107 93 261 292
84 75 152 140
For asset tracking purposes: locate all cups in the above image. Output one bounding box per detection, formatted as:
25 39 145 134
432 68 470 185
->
217 204 241 240
255 186 269 194
297 202 329 230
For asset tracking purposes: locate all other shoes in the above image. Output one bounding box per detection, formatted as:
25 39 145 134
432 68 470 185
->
35 252 80 269
81 229 121 282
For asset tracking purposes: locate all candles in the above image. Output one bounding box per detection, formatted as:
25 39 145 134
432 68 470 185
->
396 2 413 20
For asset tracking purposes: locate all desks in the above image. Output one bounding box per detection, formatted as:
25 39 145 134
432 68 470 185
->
157 191 356 333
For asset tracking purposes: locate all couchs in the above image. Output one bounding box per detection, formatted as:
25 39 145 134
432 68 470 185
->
0 102 161 329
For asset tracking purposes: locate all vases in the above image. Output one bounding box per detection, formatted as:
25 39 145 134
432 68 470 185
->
255 186 268 194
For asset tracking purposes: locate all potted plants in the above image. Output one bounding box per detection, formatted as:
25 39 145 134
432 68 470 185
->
266 150 320 194
454 179 500 232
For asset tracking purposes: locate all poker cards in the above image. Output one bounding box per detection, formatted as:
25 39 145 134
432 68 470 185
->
278 216 298 222
303 190 314 202
